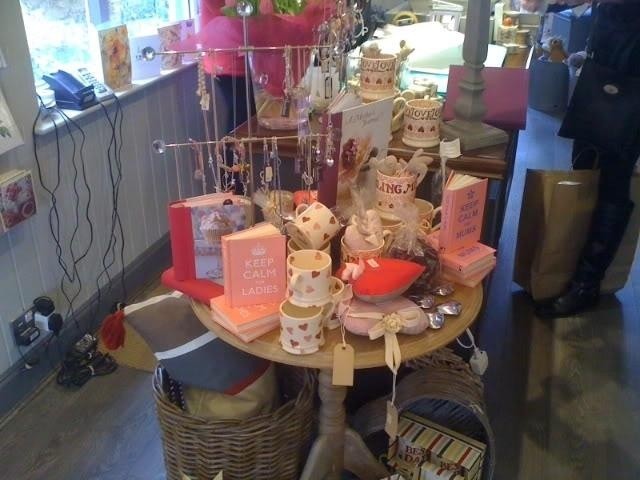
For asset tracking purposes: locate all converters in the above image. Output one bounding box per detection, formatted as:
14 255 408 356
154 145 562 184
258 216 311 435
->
19 327 40 346
71 333 97 358
34 311 58 332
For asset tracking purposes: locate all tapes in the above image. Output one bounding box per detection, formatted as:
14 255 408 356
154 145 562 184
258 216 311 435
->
393 11 418 27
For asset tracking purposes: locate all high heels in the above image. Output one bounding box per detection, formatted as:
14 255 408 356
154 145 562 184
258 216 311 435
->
534 275 601 320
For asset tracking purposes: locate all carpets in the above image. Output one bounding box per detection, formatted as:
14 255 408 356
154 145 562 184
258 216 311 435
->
96 282 173 373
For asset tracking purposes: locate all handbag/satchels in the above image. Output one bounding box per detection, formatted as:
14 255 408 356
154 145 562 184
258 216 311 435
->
514 169 640 302
557 57 640 163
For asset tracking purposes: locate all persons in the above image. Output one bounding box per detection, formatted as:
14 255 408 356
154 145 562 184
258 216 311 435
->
518 1 639 324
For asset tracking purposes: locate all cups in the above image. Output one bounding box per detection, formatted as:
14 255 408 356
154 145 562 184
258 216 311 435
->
375 166 417 216
338 230 394 265
400 99 443 150
283 200 342 254
286 248 332 309
415 196 443 235
277 276 344 358
359 53 397 102
364 209 403 232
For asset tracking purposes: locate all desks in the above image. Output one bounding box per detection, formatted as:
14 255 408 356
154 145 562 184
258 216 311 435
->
223 111 515 200
187 277 487 479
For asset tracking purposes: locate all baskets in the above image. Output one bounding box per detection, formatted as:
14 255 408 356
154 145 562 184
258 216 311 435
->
353 346 496 479
151 346 496 479
152 362 318 480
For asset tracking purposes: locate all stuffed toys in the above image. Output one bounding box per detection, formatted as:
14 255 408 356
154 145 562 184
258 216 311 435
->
566 50 592 104
540 37 568 64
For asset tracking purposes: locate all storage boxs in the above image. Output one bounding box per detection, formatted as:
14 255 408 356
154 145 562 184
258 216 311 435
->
550 12 588 56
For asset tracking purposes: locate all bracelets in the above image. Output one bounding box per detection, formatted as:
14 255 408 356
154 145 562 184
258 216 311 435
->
313 8 367 75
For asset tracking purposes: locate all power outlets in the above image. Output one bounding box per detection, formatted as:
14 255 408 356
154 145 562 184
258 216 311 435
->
10 307 54 346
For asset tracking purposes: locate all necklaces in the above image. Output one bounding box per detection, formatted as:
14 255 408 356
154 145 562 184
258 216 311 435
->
189 46 334 235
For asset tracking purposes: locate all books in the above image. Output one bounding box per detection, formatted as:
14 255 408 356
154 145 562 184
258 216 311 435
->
220 224 290 308
423 228 499 288
314 83 396 212
210 293 287 332
438 168 488 251
0 46 38 233
161 267 224 310
213 312 281 343
167 188 257 280
